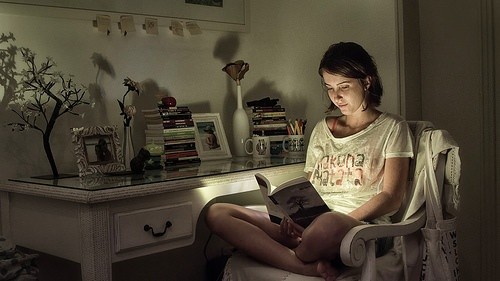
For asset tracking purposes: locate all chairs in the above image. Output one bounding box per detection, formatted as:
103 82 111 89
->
222 121 461 281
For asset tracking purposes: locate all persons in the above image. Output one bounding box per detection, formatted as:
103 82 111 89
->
204 40 416 281
203 128 220 150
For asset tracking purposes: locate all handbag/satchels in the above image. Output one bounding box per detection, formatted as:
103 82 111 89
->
421 130 460 281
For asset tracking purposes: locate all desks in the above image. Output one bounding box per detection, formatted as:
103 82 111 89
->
0 152 326 281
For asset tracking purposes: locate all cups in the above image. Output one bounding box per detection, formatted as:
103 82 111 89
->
244 137 270 157
282 135 306 155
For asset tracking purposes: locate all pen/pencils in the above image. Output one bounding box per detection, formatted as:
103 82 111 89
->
285 116 309 136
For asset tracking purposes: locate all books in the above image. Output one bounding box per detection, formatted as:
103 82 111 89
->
142 107 201 166
255 170 331 237
251 105 288 131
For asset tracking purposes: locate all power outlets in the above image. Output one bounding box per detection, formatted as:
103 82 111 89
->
196 198 216 231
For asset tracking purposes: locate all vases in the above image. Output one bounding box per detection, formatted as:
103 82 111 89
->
232 85 251 156
122 125 136 173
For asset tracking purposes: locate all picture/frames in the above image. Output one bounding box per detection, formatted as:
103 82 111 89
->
69 124 126 177
191 112 232 161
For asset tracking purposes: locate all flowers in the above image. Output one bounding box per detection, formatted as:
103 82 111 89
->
222 59 250 86
116 76 145 127
